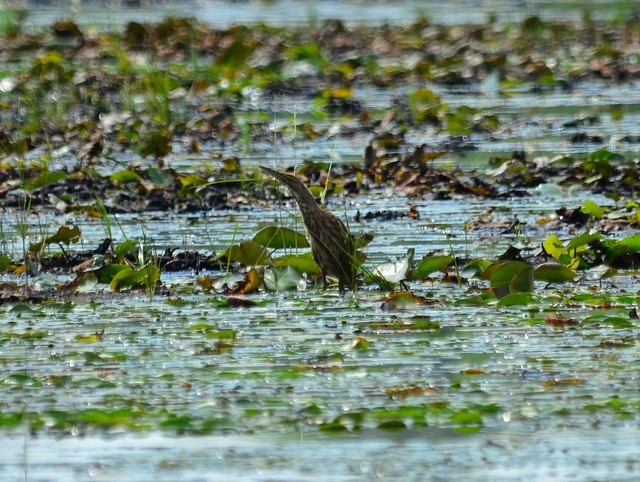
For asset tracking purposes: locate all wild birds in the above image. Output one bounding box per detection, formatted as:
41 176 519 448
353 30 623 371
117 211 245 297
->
259 166 357 296
